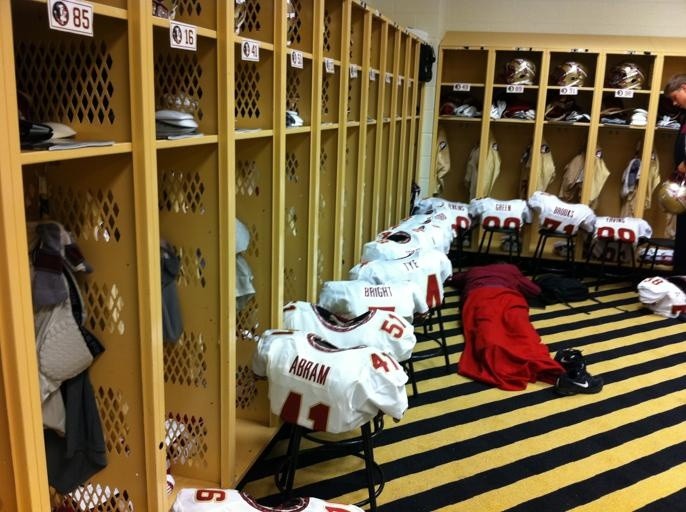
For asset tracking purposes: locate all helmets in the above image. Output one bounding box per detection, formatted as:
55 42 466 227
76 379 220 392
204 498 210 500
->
558 62 588 87
506 59 536 86
658 181 686 214
610 63 645 90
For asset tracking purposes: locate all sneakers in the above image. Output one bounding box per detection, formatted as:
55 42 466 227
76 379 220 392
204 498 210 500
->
452 234 470 247
554 348 604 396
554 246 572 257
501 240 518 252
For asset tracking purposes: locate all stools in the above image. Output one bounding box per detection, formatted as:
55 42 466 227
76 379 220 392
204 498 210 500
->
271 299 450 512
447 226 675 289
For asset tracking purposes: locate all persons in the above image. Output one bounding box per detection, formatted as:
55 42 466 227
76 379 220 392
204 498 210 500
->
663 73 686 293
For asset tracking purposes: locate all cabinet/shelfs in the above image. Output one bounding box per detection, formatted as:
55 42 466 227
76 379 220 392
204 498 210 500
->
4 0 428 512
428 29 686 269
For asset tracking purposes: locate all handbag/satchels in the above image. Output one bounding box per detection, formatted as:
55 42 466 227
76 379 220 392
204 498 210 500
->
520 277 589 306
